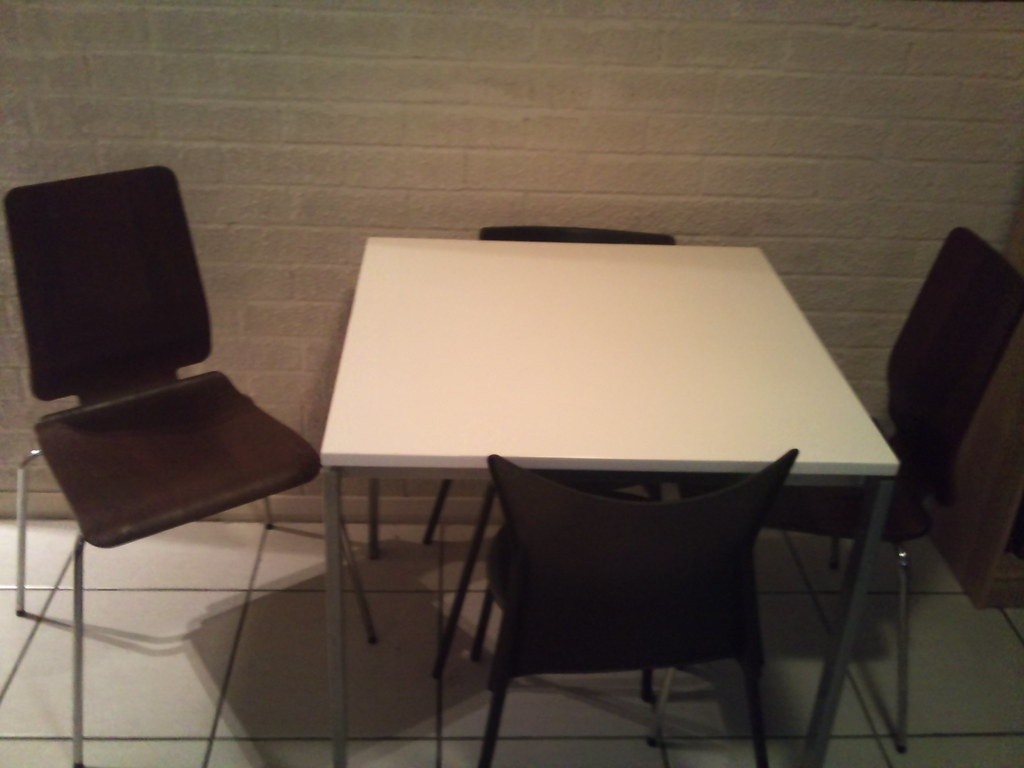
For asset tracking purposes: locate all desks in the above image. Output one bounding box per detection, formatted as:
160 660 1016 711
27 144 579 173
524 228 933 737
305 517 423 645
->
316 234 899 767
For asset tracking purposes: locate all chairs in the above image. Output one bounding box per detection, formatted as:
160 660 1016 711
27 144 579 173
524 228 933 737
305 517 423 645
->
647 226 1024 752
3 166 379 768
423 226 800 768
